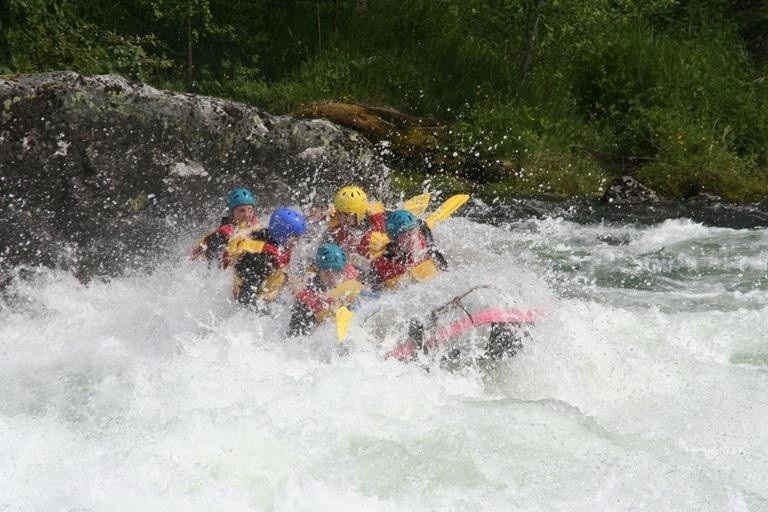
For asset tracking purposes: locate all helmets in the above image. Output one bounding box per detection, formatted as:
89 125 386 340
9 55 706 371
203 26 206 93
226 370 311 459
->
227 186 417 269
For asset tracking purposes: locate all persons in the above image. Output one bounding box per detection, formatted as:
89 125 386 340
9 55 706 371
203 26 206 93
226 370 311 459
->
191 186 447 337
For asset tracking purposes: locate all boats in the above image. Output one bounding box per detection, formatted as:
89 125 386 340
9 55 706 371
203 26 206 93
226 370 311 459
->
307 226 550 374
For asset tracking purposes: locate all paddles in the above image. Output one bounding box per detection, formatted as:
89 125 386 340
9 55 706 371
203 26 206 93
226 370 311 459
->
391 192 433 221
425 194 471 228
328 280 382 305
